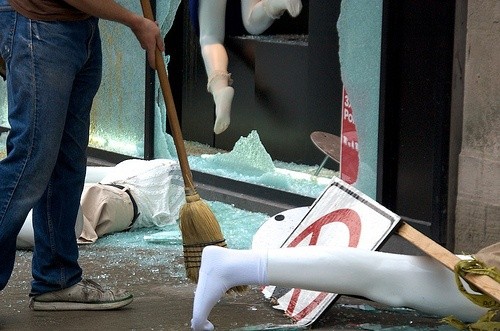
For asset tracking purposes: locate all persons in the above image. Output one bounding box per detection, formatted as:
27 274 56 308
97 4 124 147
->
0 0 163 309
198 0 302 134
192 243 500 331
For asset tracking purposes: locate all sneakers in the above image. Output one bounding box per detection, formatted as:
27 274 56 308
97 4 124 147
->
31 279 133 309
